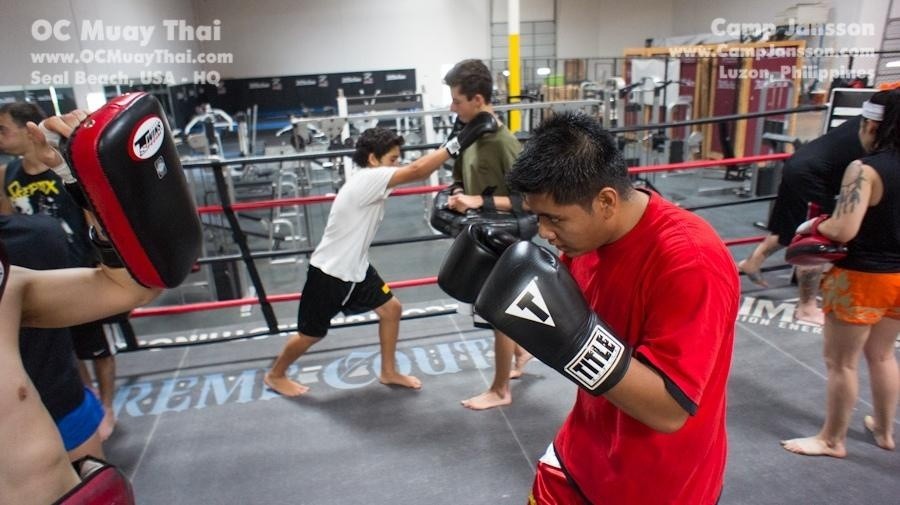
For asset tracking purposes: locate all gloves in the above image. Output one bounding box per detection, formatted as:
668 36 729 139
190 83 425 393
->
438 222 631 396
439 111 498 159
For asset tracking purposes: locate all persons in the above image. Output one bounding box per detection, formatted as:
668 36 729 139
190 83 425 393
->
444 59 530 411
264 110 498 397
736 89 900 459
1 102 164 505
437 109 742 505
